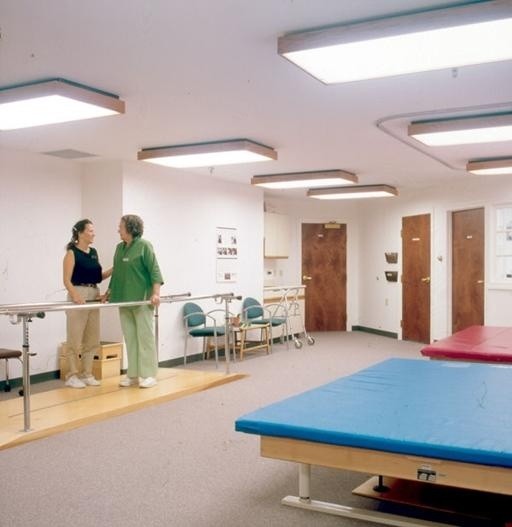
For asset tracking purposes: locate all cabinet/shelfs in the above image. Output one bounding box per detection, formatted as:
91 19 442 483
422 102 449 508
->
264 285 307 344
264 211 290 258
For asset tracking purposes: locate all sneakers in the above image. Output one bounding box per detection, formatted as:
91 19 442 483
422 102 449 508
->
138 377 157 389
79 375 101 386
119 376 138 387
63 375 86 389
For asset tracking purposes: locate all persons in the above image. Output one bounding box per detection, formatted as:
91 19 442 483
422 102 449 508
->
62 217 114 389
99 212 164 389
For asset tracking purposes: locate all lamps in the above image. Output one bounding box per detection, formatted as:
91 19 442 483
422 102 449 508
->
408 113 512 146
277 2 512 86
465 157 512 177
138 138 278 169
307 184 400 200
1 78 125 130
250 168 359 189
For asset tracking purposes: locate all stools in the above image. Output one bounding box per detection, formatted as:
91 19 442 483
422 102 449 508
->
1 346 23 397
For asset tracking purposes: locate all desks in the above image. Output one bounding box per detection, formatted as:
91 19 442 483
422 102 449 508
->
206 322 270 362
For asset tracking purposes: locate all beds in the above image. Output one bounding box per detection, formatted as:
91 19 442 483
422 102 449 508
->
420 322 511 364
234 353 511 527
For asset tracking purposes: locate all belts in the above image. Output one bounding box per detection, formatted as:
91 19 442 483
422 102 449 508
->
72 283 98 289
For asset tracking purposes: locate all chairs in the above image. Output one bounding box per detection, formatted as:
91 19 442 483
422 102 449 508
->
181 302 241 373
242 296 289 354
